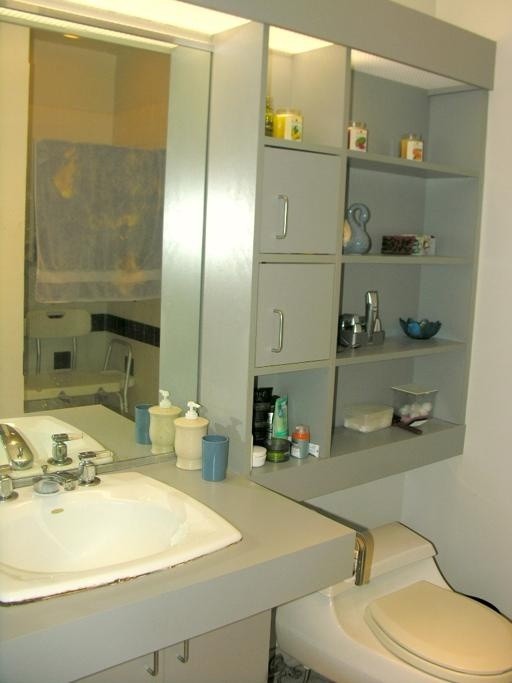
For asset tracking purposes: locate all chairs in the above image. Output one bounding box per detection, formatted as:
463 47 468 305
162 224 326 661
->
27 307 91 376
99 339 134 418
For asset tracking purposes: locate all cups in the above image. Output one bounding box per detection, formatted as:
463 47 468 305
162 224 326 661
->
134 403 154 446
201 434 230 482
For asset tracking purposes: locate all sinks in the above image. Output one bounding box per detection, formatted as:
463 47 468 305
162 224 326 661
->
0 415 114 477
1 469 210 573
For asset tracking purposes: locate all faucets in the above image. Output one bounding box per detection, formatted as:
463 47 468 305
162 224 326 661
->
53 471 79 492
0 422 33 484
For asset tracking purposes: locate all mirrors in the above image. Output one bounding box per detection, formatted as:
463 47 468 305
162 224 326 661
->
0 1 214 487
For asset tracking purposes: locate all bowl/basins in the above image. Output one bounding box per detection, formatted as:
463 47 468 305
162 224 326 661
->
397 316 442 340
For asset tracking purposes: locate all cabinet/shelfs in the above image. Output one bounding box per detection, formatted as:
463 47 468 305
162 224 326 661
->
72 608 272 683
192 2 495 500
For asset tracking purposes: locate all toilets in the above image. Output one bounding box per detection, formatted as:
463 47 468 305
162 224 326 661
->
274 522 512 683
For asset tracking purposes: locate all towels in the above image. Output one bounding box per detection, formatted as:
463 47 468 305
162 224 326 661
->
25 140 166 305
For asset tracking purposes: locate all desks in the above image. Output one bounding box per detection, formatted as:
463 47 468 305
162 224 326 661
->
24 368 123 412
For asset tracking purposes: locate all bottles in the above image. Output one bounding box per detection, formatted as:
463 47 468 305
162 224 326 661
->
399 132 424 163
348 120 368 153
273 108 303 142
264 95 272 137
